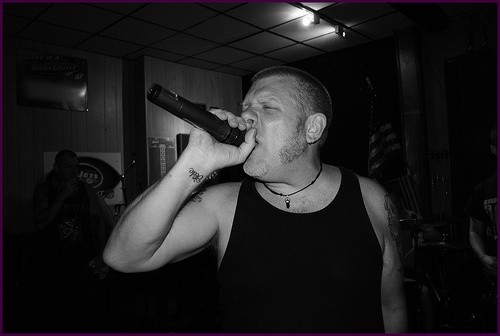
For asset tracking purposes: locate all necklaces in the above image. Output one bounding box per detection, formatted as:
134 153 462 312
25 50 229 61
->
259 161 322 209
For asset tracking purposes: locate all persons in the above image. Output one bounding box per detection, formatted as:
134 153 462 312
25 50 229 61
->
101 65 414 336
465 127 497 332
30 147 118 263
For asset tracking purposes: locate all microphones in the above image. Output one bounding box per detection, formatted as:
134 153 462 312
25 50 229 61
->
147 84 246 148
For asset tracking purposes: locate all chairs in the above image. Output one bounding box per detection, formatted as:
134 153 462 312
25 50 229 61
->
386 175 449 254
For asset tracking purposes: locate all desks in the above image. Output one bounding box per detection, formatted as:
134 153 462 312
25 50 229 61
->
400 209 425 230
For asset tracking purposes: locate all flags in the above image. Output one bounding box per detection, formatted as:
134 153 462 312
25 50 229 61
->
362 85 401 176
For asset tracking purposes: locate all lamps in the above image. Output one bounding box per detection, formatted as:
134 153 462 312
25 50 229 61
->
300 13 320 28
333 24 347 39
